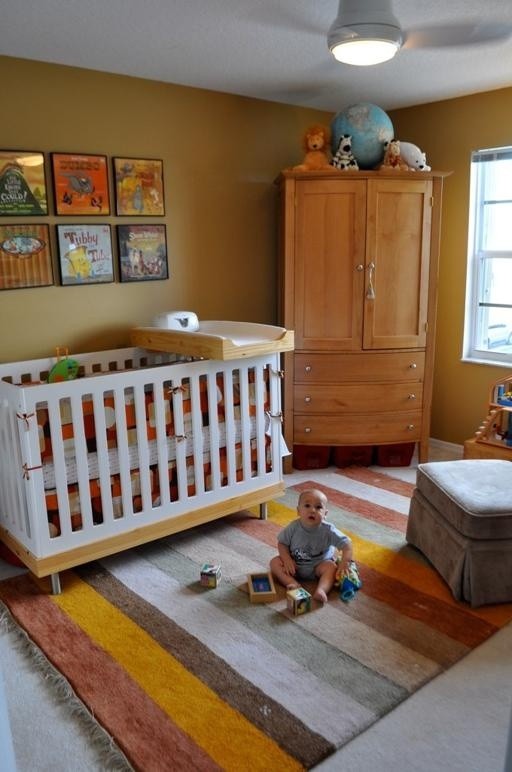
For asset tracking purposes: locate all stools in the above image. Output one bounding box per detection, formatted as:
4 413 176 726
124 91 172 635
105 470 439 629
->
405 459 512 608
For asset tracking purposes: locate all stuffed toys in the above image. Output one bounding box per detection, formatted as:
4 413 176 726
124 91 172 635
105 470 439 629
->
329 132 360 170
292 121 337 171
400 141 433 172
380 138 409 173
46 345 81 384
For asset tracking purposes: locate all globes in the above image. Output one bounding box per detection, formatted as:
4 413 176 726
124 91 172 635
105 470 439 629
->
330 102 394 169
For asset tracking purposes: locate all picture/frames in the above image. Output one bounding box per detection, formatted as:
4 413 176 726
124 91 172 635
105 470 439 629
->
56 223 115 286
0 149 49 216
0 223 54 290
117 224 169 282
49 152 111 216
112 156 165 217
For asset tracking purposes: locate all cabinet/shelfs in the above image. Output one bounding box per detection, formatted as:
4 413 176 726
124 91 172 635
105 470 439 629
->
283 350 435 474
273 172 451 349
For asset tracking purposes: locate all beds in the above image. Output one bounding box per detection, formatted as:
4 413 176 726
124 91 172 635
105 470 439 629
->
0 346 286 595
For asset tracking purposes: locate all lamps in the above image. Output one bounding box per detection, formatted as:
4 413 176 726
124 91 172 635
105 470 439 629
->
326 0 405 68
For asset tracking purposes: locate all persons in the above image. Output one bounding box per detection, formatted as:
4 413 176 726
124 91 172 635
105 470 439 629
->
269 487 353 605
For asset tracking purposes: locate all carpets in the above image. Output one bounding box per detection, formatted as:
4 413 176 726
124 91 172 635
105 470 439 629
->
1 458 510 772
